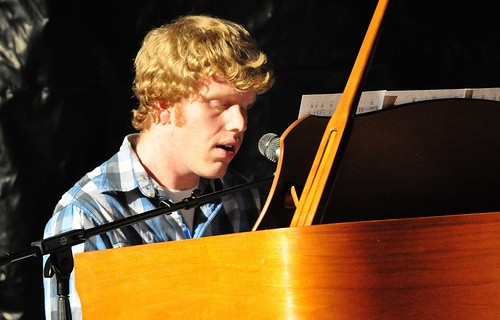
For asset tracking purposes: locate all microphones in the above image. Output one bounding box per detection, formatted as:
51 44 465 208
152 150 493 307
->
258 133 280 162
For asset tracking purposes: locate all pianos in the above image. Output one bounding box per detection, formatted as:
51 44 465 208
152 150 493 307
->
68 1 500 320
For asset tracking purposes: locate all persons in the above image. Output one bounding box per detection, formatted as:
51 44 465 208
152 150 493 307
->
40 14 281 320
